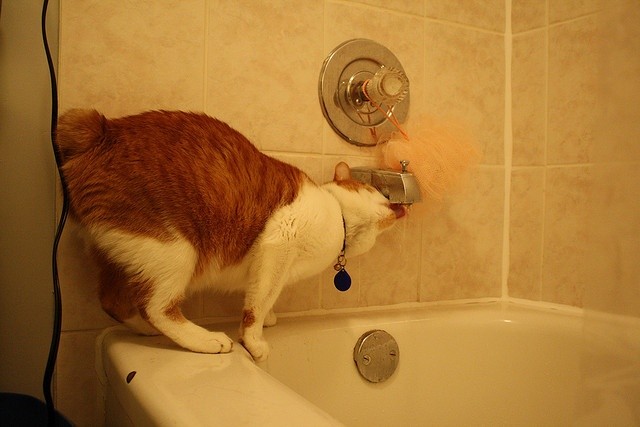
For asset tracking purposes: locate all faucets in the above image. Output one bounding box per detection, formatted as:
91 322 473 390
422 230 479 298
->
350 159 420 205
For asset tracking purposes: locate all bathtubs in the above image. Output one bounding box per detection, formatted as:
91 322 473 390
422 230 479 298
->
102 299 640 427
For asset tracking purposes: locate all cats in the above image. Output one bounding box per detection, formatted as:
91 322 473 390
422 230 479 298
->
55 108 414 365
